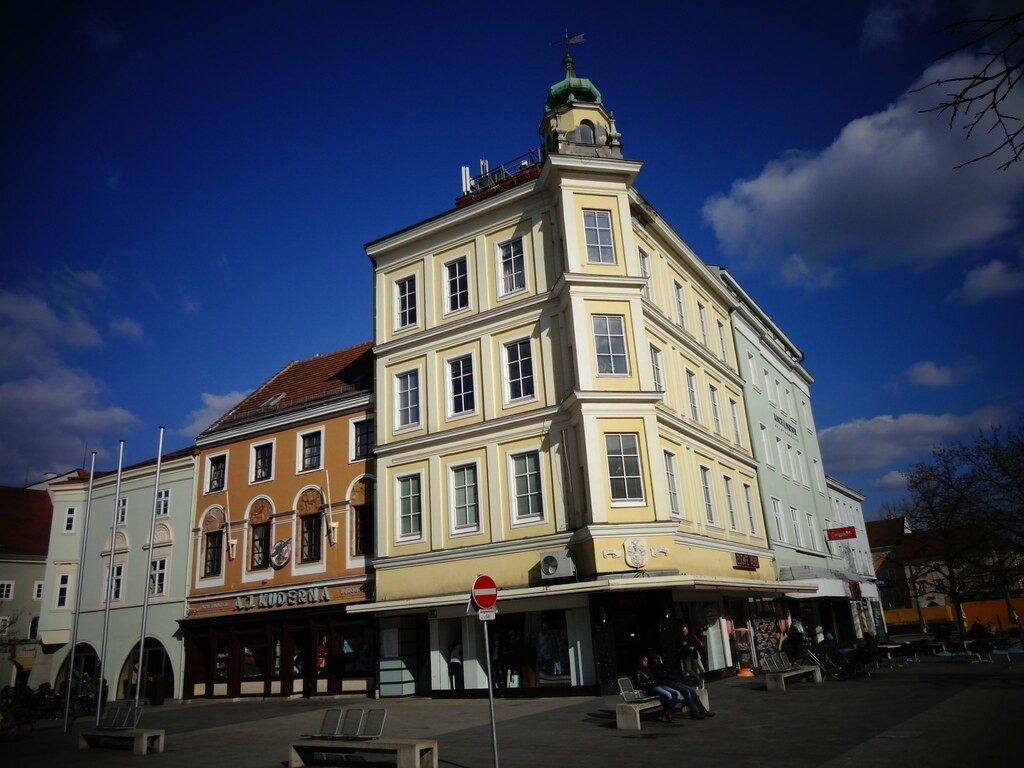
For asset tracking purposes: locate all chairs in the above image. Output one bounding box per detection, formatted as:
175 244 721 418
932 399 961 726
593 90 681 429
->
807 633 1024 682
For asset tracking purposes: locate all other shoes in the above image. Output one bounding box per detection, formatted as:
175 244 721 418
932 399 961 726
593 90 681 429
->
661 711 674 723
691 713 704 719
701 710 715 717
695 685 698 690
701 679 705 689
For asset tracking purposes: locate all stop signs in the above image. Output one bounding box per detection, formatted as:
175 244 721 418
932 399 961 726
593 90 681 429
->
474 576 500 610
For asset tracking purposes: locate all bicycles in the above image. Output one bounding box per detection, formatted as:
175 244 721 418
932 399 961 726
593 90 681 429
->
791 641 850 683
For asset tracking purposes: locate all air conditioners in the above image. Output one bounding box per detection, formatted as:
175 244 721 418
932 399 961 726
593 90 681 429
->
540 549 577 579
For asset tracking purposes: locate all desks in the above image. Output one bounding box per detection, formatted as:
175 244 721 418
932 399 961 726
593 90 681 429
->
963 641 988 664
876 645 904 669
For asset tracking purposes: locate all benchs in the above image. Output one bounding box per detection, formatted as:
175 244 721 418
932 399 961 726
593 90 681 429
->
288 707 438 768
764 652 822 691
615 676 710 730
78 706 165 755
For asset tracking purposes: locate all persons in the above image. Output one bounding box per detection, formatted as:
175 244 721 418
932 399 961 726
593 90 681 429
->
970 617 996 638
631 627 716 722
